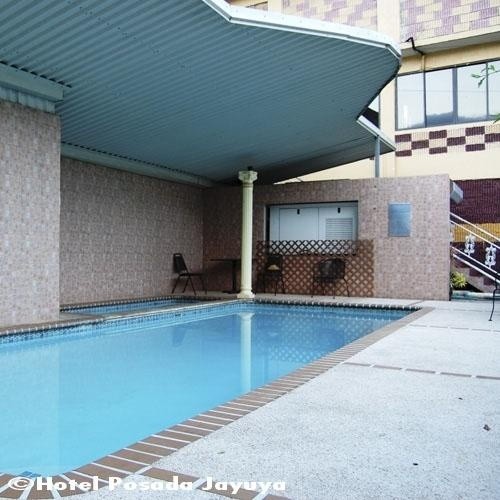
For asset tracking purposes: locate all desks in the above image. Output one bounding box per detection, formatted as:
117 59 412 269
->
211 256 255 294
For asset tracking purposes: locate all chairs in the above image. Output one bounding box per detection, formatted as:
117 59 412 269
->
488 272 500 320
257 254 284 294
170 253 207 296
311 257 351 298
